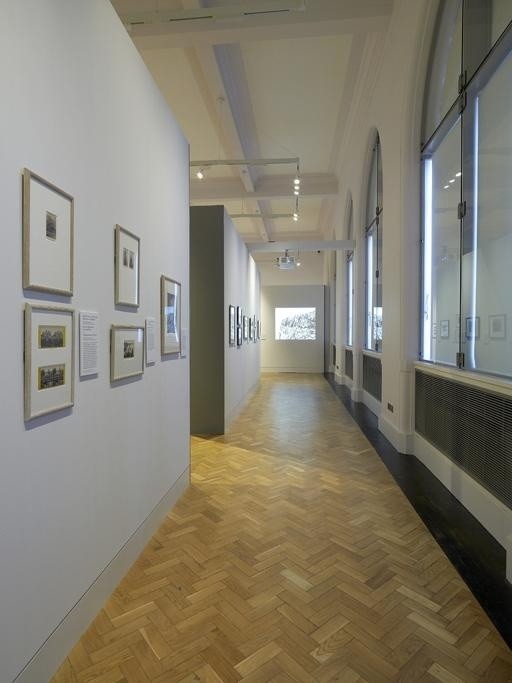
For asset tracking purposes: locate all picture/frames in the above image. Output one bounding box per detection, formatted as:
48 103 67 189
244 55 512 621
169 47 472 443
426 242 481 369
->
228 305 261 347
113 223 141 308
24 168 76 427
111 324 146 383
159 275 183 357
